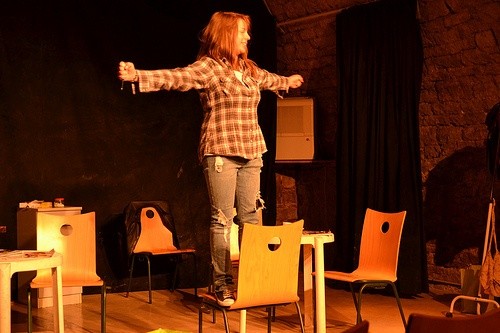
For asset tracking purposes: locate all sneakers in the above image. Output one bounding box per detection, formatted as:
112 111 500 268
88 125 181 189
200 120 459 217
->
215 289 234 306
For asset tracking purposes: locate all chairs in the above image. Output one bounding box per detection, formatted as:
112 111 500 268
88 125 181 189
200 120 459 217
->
310 208 408 332
27 212 108 333
198 219 305 333
123 199 198 305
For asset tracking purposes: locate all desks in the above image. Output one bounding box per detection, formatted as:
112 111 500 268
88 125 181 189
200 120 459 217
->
266 230 333 333
0 247 68 333
16 200 83 308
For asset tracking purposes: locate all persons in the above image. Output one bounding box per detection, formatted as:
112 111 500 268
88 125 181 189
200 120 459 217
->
117 11 303 306
